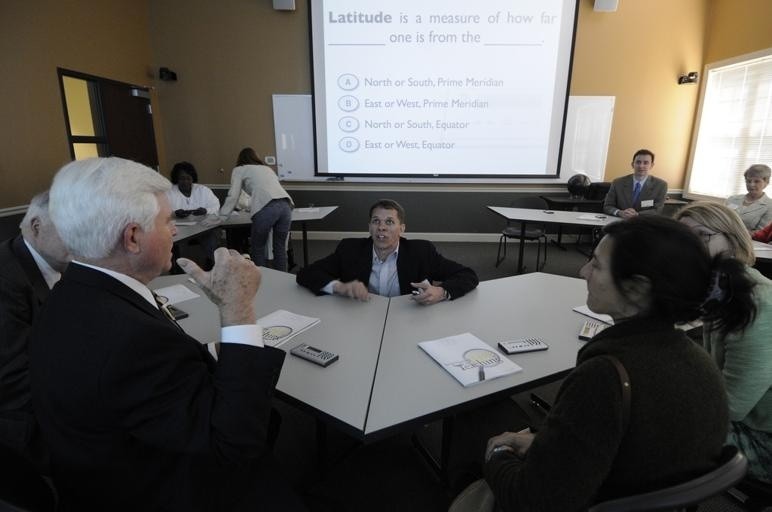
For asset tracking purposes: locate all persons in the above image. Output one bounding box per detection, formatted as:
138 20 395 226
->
750 222 772 281
443 209 760 512
297 198 479 306
722 164 772 231
671 199 772 512
0 188 64 512
587 148 668 263
28 155 288 512
219 148 294 272
169 161 221 275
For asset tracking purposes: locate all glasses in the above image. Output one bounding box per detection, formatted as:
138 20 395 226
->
696 228 724 242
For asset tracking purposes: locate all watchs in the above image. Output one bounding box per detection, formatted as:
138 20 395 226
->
484 445 516 462
444 290 451 301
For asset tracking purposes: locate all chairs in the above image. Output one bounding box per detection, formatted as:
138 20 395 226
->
496 199 552 270
586 445 751 512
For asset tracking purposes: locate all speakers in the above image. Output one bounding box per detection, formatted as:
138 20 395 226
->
273 1 297 10
592 1 619 13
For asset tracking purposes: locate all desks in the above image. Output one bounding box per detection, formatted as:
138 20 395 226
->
364 270 704 445
751 239 772 262
174 206 340 269
170 218 220 243
148 266 391 434
486 204 626 272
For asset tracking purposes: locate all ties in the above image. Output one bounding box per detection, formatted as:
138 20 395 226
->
632 181 642 202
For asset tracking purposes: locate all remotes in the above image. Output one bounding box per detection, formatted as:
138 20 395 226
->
499 337 548 354
291 341 338 368
580 320 599 338
